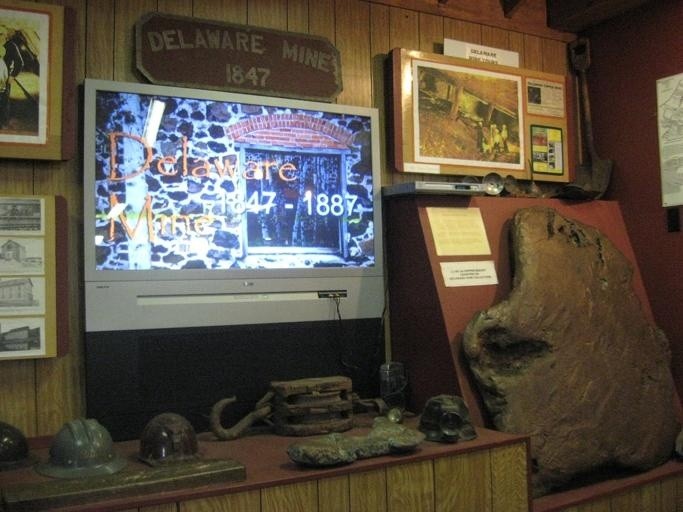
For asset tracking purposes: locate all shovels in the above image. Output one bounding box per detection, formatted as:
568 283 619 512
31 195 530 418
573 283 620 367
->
569 37 613 200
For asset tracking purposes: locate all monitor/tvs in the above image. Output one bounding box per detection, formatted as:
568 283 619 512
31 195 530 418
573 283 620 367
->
84 79 385 331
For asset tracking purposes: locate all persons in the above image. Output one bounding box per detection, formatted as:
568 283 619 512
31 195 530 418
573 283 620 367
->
474 120 508 160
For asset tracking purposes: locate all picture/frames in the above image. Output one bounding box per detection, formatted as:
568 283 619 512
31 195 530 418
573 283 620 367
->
0 0 67 165
